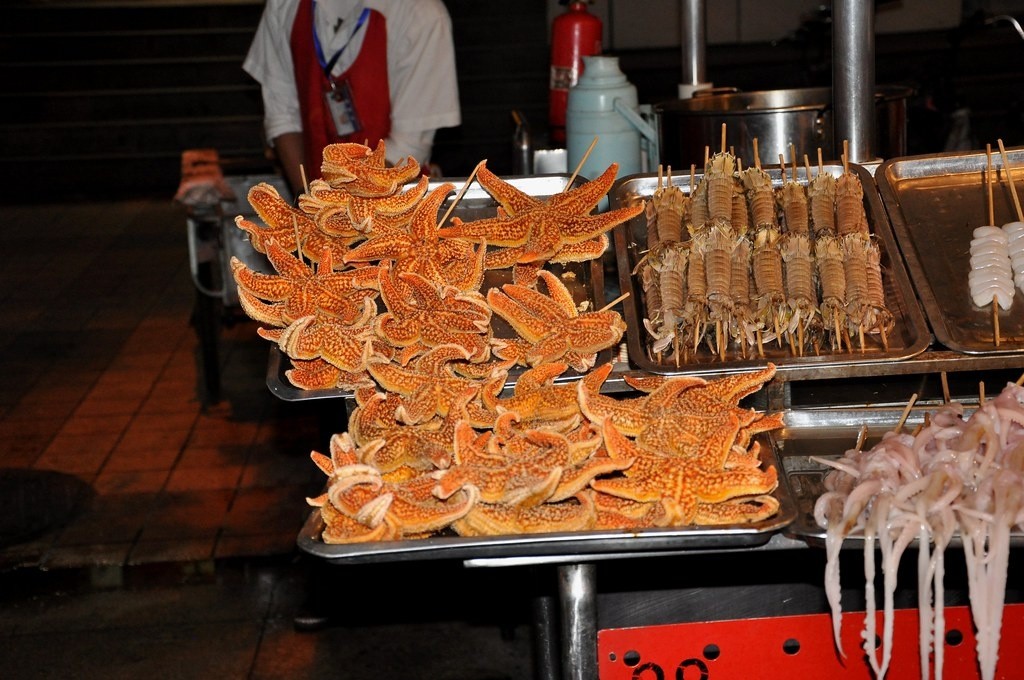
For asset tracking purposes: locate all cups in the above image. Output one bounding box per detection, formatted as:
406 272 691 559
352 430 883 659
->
534 150 568 176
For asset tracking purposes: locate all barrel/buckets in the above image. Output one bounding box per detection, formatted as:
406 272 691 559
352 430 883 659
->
174 177 288 318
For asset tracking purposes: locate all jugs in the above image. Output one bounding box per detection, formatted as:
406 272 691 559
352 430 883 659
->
564 56 658 214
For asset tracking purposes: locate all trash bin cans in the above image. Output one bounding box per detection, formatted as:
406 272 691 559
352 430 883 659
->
875 85 913 159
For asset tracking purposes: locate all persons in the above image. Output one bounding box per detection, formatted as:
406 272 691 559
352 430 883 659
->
243 1 461 201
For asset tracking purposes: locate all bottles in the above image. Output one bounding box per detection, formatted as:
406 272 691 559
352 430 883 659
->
550 0 602 138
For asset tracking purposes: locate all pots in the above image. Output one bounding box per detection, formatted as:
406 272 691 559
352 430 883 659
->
682 87 914 171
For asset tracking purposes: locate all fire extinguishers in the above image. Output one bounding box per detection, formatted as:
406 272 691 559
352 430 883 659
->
549 0 611 142
